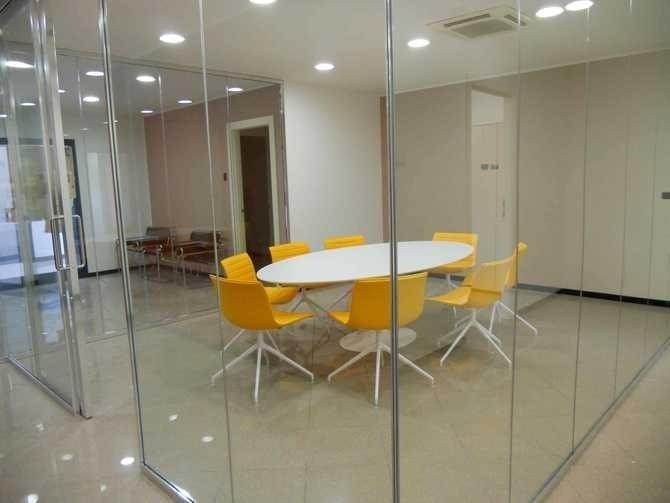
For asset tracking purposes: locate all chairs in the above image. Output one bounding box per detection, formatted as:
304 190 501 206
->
430 231 481 321
426 253 513 369
327 272 435 407
208 275 316 408
324 236 368 310
116 225 223 287
269 241 333 339
454 242 538 341
220 253 302 359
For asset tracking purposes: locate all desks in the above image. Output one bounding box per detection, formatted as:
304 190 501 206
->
255 239 472 353
